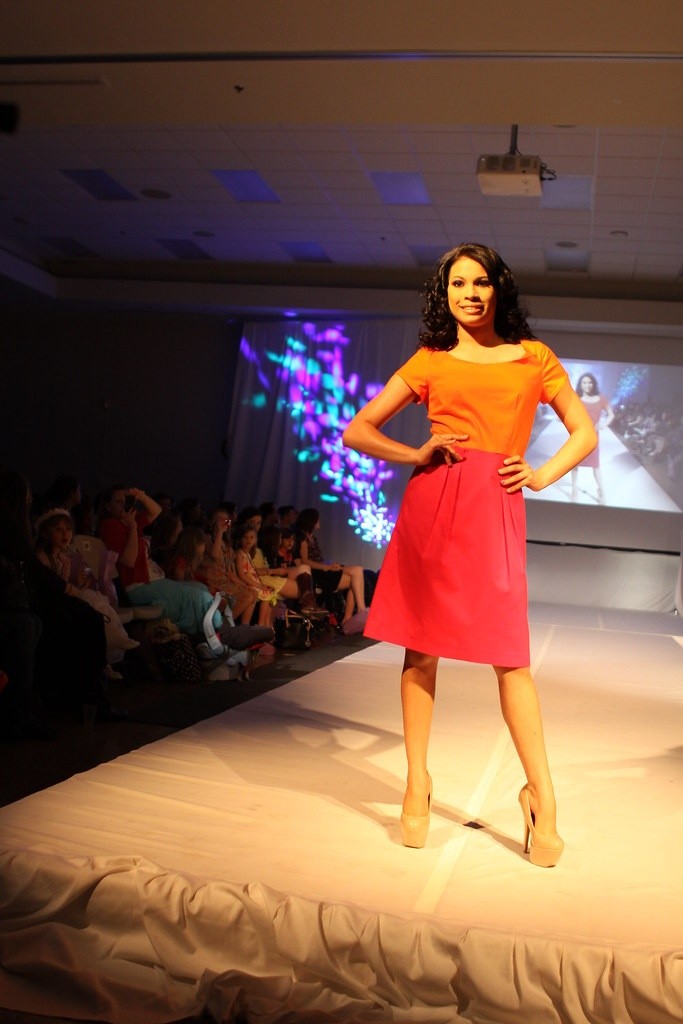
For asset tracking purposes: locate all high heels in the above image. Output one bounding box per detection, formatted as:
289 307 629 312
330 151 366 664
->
401 772 433 847
519 784 563 867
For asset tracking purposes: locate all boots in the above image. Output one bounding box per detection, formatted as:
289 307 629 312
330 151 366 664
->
296 572 330 615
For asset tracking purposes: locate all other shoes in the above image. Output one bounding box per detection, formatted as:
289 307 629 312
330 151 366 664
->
106 665 123 680
118 636 140 649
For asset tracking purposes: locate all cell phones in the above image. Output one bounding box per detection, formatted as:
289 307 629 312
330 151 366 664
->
223 518 232 526
124 493 135 512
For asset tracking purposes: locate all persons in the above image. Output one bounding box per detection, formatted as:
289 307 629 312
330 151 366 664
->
609 398 683 483
569 373 614 505
342 244 599 867
0 457 371 721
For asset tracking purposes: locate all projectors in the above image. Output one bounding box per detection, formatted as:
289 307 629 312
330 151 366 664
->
476 123 542 197
